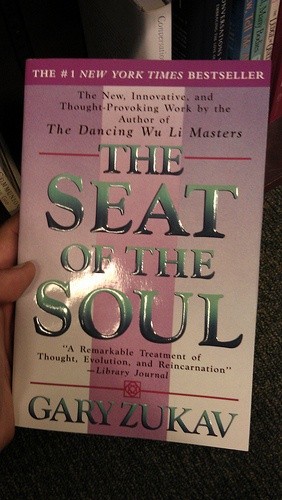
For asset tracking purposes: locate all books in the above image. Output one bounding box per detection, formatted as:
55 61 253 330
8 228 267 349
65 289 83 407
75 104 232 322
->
0 0 281 217
11 57 274 450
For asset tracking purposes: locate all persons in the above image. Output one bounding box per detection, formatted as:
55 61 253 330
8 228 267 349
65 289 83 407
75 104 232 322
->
1 211 36 443
